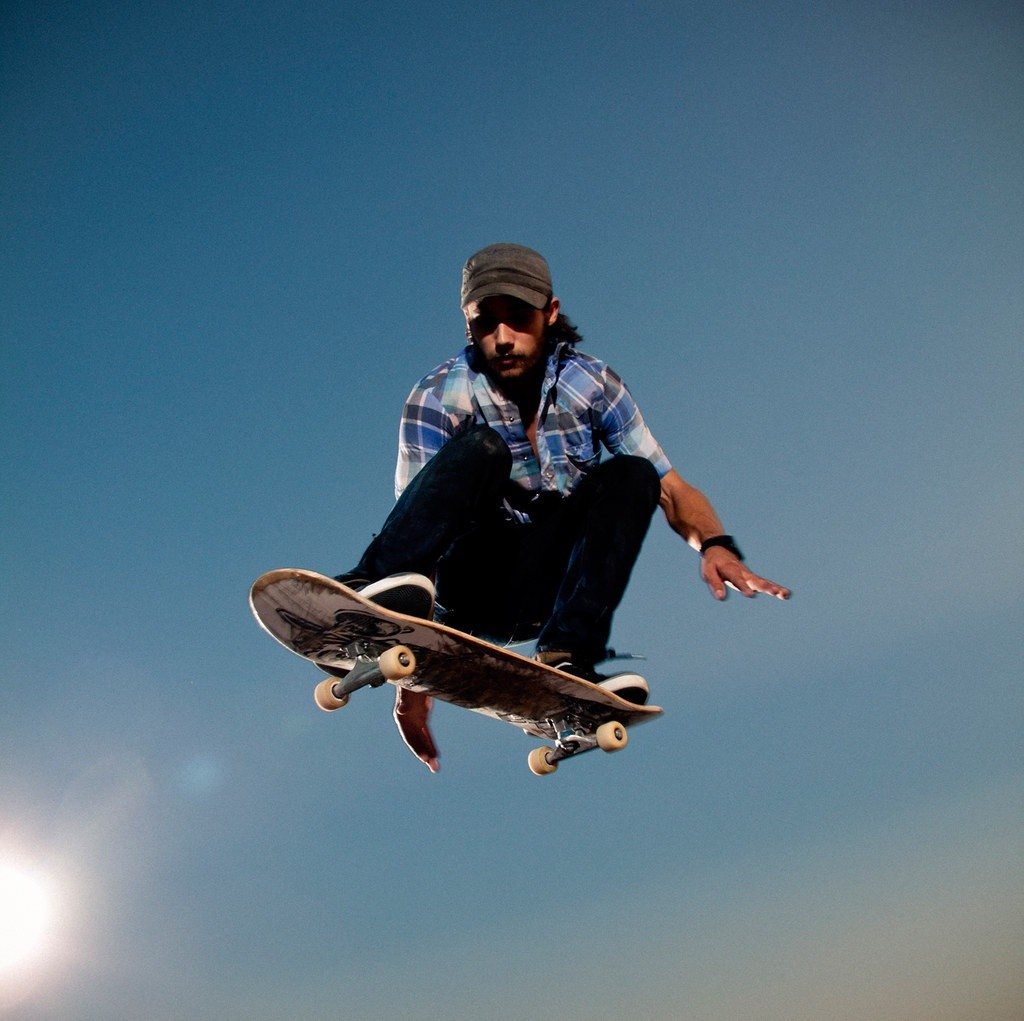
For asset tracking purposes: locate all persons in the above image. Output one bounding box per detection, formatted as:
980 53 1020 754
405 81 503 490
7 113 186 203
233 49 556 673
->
333 243 795 775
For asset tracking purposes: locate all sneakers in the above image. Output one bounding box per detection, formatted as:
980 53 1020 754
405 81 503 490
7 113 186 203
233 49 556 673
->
552 663 649 706
314 571 436 680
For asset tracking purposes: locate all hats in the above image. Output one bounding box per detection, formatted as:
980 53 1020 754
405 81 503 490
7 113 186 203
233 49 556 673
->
460 242 552 310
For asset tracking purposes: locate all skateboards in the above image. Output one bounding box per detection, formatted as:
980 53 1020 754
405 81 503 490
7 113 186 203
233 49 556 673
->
248 568 666 776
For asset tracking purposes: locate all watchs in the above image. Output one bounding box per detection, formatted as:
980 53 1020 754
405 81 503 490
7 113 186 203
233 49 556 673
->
700 535 744 561
